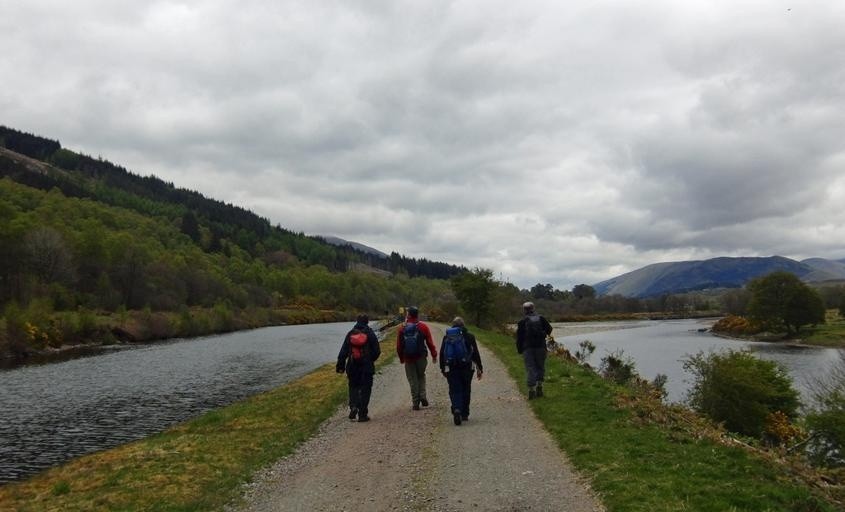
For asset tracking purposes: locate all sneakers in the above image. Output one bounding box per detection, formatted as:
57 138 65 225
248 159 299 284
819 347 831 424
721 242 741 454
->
453 409 469 425
412 405 419 410
420 398 429 407
348 408 358 419
528 384 543 400
357 416 370 422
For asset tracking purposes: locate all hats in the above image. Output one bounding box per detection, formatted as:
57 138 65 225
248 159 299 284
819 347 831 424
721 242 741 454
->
406 306 419 313
522 301 536 313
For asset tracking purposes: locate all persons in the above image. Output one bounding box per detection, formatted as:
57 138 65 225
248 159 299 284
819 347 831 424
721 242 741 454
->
335 311 381 423
439 315 485 426
515 301 553 402
396 306 437 412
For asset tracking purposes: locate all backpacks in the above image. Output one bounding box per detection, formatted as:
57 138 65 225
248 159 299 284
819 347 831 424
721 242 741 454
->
401 321 422 359
350 328 371 366
440 326 474 379
525 315 546 347
